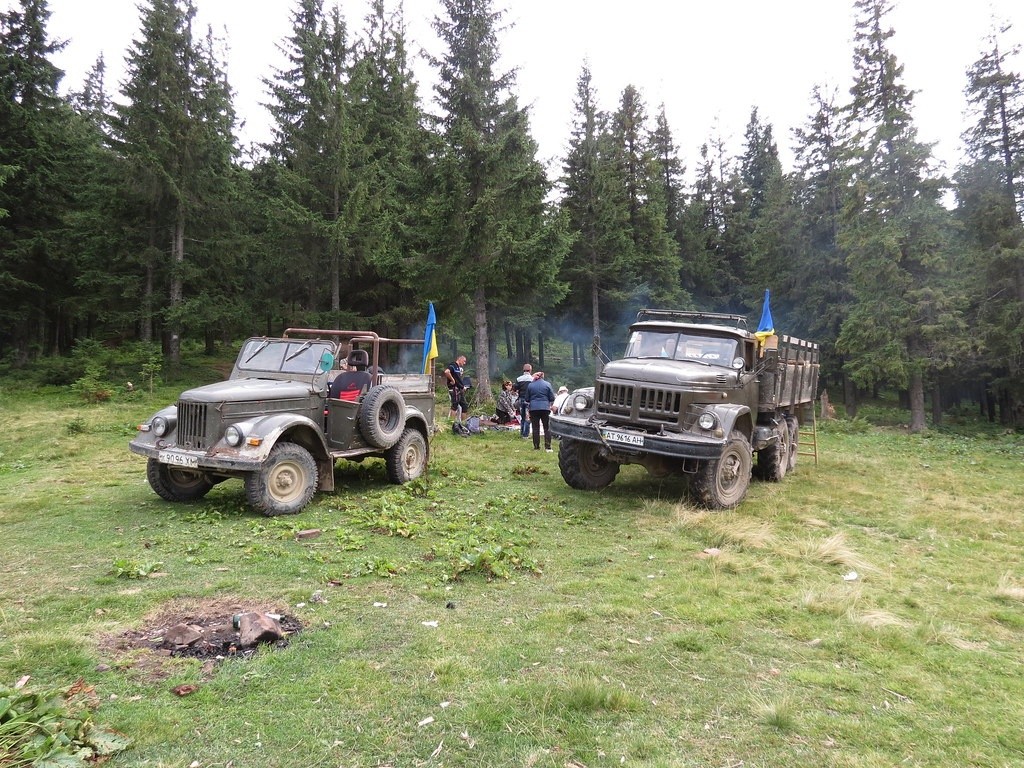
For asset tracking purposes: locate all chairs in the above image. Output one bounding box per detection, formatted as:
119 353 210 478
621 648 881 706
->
324 349 373 418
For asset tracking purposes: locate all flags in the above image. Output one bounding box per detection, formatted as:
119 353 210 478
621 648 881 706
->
755 290 775 346
420 304 439 374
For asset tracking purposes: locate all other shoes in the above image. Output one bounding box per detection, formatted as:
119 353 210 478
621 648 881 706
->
545 449 555 453
533 447 541 450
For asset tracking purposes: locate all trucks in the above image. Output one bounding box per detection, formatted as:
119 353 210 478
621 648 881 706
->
547 312 820 511
130 325 438 518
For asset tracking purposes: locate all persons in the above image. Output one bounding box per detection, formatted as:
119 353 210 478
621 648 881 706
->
490 380 519 424
340 358 353 370
551 385 569 440
512 363 533 437
444 355 467 420
524 372 555 452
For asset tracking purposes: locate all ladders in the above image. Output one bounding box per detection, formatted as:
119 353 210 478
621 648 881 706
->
797 401 819 466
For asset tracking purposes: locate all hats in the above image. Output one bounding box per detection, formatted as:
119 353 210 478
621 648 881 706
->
557 386 569 392
522 363 532 371
